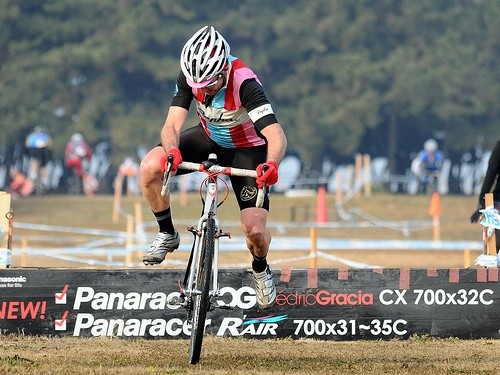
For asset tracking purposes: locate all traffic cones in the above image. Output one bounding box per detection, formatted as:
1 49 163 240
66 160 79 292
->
428 192 440 217
315 187 328 223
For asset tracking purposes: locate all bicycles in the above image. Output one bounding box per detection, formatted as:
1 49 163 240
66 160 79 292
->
159 153 270 366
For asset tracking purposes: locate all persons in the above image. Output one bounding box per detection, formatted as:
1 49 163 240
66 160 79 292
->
140 25 287 310
471 145 500 254
5 122 91 199
411 139 445 185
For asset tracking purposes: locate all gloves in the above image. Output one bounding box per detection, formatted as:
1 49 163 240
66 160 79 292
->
160 148 183 172
471 207 488 222
254 160 278 188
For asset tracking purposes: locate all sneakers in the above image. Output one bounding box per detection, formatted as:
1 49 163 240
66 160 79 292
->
142 230 180 266
251 260 277 308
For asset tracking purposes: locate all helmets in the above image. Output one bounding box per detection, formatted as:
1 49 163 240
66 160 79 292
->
423 138 439 153
180 26 231 88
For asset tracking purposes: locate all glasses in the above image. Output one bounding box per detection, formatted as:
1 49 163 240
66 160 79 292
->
205 70 224 88
